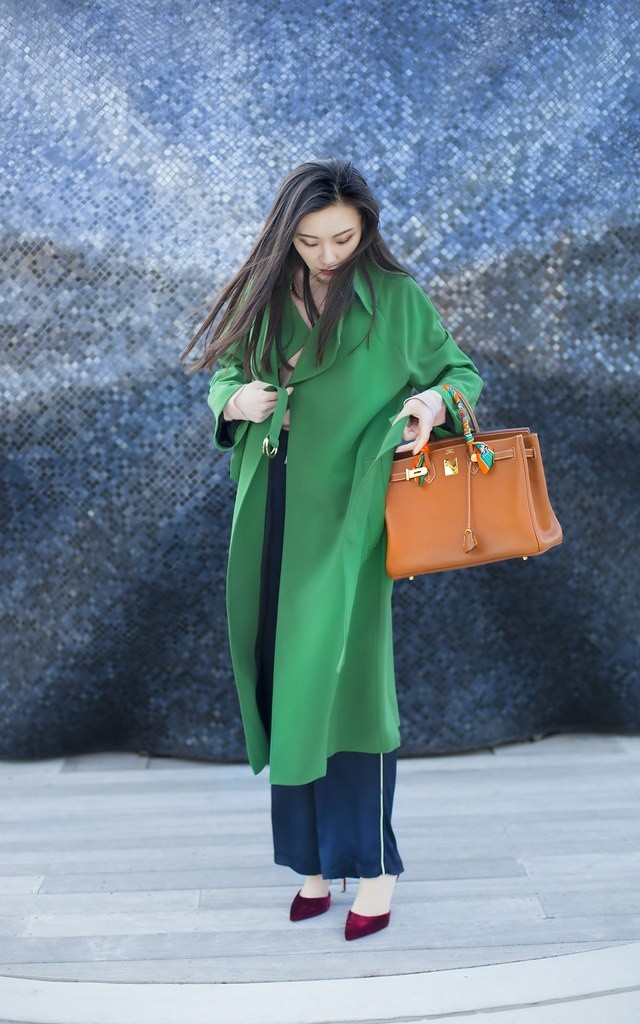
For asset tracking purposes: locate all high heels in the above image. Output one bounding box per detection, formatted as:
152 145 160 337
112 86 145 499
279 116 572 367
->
290 876 345 922
344 868 399 941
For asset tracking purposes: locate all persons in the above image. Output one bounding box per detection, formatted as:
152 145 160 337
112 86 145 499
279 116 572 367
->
199 162 487 943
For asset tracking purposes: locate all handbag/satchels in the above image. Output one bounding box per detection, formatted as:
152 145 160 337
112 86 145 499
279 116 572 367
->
385 383 562 581
